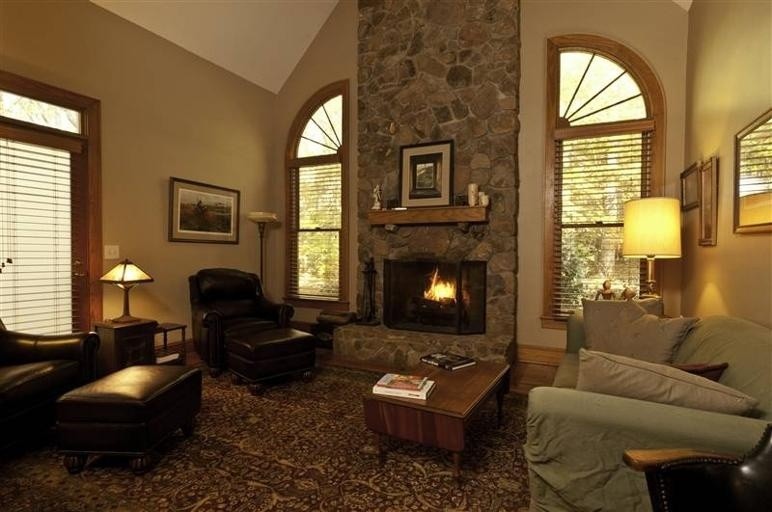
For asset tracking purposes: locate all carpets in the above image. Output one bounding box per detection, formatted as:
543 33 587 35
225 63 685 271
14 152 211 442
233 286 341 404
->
0 361 532 511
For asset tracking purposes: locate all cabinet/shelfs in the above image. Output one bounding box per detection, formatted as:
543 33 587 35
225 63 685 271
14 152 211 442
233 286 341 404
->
95 316 160 371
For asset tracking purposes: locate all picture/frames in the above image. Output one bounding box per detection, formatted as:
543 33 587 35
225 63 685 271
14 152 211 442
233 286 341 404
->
679 159 700 211
697 154 717 246
167 176 241 245
398 138 454 207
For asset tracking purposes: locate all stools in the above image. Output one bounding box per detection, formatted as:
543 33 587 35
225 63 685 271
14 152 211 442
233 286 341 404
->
226 327 317 396
52 364 202 476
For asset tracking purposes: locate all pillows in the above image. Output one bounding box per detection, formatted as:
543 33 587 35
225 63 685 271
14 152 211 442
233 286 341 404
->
580 296 665 349
668 359 729 383
588 297 702 367
574 346 761 418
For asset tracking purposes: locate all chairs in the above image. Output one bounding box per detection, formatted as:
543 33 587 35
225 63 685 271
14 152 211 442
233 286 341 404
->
186 267 296 379
620 416 772 512
0 318 101 466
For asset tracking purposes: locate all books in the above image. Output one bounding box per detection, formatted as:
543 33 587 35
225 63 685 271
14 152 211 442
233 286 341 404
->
372 372 435 401
420 349 477 372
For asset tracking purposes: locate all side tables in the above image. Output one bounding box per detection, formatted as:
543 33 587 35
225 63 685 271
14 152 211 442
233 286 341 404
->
153 320 187 366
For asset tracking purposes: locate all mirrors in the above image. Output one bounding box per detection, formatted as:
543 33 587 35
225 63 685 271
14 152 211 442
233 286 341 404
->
732 107 772 235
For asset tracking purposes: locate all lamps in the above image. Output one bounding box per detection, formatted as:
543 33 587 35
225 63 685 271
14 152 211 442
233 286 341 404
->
246 210 281 289
621 195 683 317
96 256 155 324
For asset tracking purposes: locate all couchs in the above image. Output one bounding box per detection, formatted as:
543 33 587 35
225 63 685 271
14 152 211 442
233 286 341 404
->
521 309 771 511
